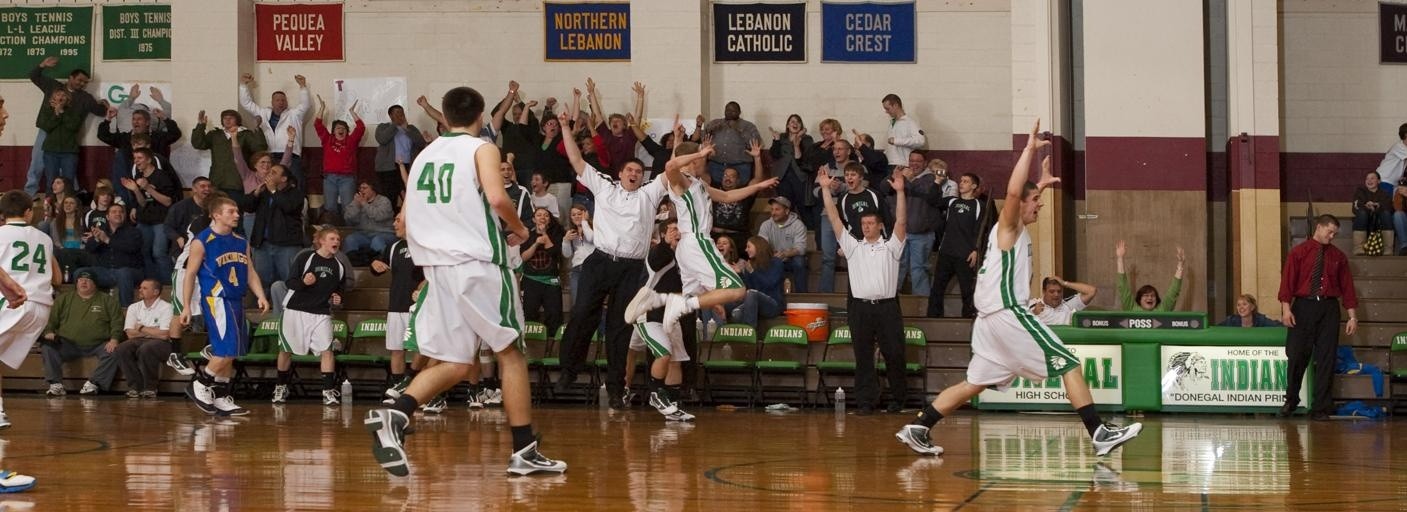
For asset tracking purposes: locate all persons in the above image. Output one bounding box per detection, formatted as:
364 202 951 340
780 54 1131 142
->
168 195 234 374
770 115 813 226
115 84 172 133
493 80 537 164
1278 214 1358 418
89 178 120 208
626 144 780 332
924 168 985 316
701 137 764 257
713 234 742 327
177 197 268 415
1352 172 1395 255
41 267 120 397
882 92 925 177
397 156 409 193
756 197 809 293
418 80 519 145
362 88 566 477
554 103 683 412
638 220 697 420
500 160 532 316
76 206 140 315
98 107 180 164
1 191 60 428
1115 240 1187 327
890 150 942 302
230 122 297 244
239 72 312 203
528 77 608 163
561 203 605 345
36 88 82 192
835 160 888 242
531 117 557 181
43 178 68 222
696 102 760 197
893 118 1142 458
374 103 423 202
368 210 430 406
728 238 786 338
0 468 34 492
83 191 113 253
127 130 181 219
812 119 854 241
1 99 9 137
524 168 558 226
245 164 306 294
818 162 908 415
632 82 705 179
555 115 606 203
48 196 88 283
123 149 180 284
26 57 108 210
848 126 887 193
165 175 208 275
342 183 392 271
520 210 563 341
923 159 952 243
192 108 264 230
317 95 363 229
1213 294 1276 327
1392 124 1407 253
815 139 867 298
115 279 171 403
587 79 646 180
272 225 345 405
1029 275 1096 324
119 147 176 289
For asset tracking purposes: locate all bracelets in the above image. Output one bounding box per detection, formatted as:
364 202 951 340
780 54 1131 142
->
287 140 294 145
972 247 979 254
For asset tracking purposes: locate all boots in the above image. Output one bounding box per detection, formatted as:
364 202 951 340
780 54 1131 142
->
1380 229 1393 256
1351 231 1367 255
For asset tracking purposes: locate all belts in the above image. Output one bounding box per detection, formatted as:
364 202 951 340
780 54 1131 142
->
707 158 753 170
592 247 647 267
848 296 901 306
574 190 591 197
1294 295 1339 302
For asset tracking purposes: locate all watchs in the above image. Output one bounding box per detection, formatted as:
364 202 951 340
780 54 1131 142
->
1347 316 1360 324
268 189 279 197
507 88 515 94
136 325 143 335
695 123 703 130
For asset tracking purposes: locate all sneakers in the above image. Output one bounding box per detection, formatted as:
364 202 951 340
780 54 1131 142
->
386 375 414 399
47 383 67 396
630 394 639 404
477 387 487 403
211 392 251 417
364 405 409 477
1398 247 1407 256
647 389 679 418
1 410 12 430
621 389 633 408
466 390 480 409
183 378 217 414
81 380 98 395
421 393 447 413
482 388 506 409
663 405 695 424
125 388 139 399
323 387 341 406
1 469 37 494
381 394 392 405
1090 421 1142 456
139 386 159 397
508 437 567 476
895 421 944 454
166 351 195 374
273 382 290 405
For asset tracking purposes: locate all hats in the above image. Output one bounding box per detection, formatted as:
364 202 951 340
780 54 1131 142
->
74 267 98 279
767 195 792 209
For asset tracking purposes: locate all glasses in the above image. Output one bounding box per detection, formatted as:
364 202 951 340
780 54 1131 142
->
908 157 926 165
74 276 93 284
544 120 558 127
831 146 850 152
721 172 737 180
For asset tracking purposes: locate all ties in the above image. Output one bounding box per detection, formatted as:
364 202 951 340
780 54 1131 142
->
1310 246 1326 300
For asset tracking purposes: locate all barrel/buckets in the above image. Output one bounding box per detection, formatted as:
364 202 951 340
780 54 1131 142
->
785 302 830 342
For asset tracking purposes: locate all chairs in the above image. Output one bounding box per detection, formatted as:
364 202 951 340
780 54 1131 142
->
234 318 311 401
288 321 347 406
703 325 757 410
814 325 877 410
877 327 927 409
185 319 253 399
1389 332 1407 417
594 327 650 407
538 324 601 407
755 325 808 411
524 321 550 403
336 320 392 397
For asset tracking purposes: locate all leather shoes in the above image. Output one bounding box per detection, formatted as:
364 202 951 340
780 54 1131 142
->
886 400 904 414
607 395 627 413
855 401 875 416
1309 410 1330 422
551 372 580 395
1278 398 1300 417
